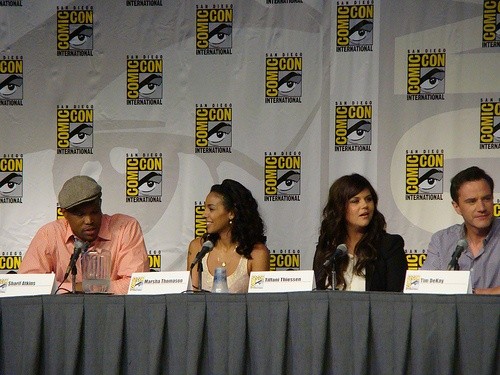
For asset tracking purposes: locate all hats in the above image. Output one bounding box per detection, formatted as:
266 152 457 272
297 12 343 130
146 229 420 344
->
58 175 102 209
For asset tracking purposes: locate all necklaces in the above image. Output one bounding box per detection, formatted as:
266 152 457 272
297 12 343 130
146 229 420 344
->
212 245 237 267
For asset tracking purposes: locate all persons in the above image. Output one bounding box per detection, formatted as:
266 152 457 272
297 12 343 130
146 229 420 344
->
188 178 270 294
313 173 408 292
18 175 150 295
420 166 500 294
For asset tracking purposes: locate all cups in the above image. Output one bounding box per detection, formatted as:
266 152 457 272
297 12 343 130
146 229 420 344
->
212 267 231 294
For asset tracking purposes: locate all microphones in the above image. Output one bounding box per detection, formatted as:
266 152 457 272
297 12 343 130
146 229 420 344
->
448 240 468 270
190 240 213 269
64 240 86 279
323 243 348 268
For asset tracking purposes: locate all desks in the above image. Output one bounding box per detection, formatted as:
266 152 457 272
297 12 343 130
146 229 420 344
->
0 291 500 375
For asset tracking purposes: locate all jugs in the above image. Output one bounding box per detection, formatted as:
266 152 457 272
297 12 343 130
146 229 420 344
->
81 249 111 293
